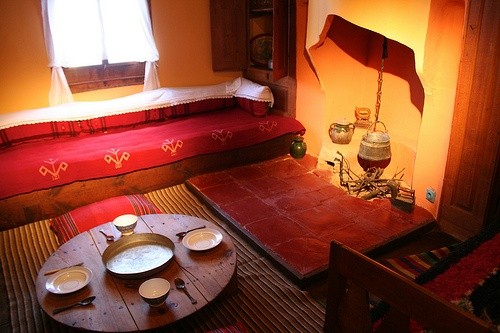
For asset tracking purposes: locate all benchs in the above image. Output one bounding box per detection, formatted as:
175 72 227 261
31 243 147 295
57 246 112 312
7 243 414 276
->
1 76 305 234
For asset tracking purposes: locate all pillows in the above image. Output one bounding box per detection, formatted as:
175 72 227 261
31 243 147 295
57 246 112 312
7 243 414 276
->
50 194 163 245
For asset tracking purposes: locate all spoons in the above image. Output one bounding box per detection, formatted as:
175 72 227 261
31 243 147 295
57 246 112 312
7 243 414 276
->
99 231 114 241
52 296 96 315
174 277 197 304
176 225 206 237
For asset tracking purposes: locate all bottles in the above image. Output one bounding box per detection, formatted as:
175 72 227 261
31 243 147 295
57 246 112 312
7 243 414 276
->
289 136 307 159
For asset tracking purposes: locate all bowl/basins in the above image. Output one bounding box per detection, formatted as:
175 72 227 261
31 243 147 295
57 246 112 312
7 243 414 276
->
112 214 138 235
328 122 355 144
139 278 171 307
101 233 175 277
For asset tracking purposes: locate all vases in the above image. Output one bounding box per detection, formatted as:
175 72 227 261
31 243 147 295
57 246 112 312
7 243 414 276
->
291 136 306 159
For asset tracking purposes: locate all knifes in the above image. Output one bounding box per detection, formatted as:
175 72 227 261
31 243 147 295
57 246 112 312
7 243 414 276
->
44 262 84 276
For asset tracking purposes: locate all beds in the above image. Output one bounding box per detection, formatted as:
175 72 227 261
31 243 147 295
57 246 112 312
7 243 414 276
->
323 218 500 333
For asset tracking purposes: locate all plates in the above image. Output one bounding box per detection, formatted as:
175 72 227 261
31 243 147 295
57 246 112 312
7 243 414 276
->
45 266 94 295
249 33 273 64
182 228 223 251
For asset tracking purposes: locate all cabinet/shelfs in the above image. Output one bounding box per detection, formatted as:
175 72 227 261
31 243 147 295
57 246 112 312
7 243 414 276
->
208 0 289 82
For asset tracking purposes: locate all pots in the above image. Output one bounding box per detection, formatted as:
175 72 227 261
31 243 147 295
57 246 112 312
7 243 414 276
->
357 121 392 171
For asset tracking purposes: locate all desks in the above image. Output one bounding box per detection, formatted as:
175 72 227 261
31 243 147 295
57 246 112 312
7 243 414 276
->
36 214 236 333
